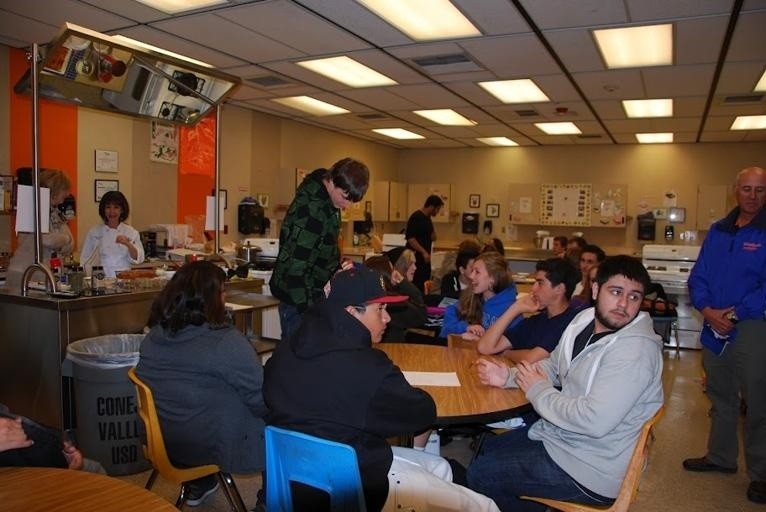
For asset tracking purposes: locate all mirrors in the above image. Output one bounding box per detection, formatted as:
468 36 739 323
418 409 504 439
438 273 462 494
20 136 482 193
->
13 21 244 129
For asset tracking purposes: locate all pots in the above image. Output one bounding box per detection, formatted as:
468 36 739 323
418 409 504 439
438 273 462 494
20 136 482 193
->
236 240 262 265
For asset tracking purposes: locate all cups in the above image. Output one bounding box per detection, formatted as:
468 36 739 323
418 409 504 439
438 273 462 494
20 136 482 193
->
535 230 550 248
184 254 196 263
82 276 91 296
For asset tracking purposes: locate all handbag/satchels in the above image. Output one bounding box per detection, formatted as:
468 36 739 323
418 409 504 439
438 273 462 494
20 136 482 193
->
423 294 444 306
640 283 678 317
0 414 69 468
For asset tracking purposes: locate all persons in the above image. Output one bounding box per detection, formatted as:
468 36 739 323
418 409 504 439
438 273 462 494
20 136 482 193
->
135 254 272 512
0 396 110 480
476 237 611 366
464 253 664 512
79 189 147 283
406 195 444 294
678 165 765 506
269 158 369 342
246 261 505 512
4 166 75 293
362 236 522 348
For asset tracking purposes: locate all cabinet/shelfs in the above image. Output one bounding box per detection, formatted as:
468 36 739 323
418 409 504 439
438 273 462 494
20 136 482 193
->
224 291 281 355
340 179 458 224
506 182 628 230
695 184 739 233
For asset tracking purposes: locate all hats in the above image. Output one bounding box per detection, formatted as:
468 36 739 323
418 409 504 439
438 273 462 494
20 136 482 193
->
329 268 410 309
699 317 736 358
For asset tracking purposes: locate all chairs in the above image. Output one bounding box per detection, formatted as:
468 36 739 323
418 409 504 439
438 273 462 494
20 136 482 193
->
519 404 665 512
128 367 248 512
264 425 367 512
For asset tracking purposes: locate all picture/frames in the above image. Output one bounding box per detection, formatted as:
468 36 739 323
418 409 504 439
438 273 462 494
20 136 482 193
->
257 192 270 209
486 203 499 218
469 194 481 208
651 207 668 219
94 178 119 202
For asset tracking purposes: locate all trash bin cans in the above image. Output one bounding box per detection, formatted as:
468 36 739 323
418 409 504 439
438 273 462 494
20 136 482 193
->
66 333 154 476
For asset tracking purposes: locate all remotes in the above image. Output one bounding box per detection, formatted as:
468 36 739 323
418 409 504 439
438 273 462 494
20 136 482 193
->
48 292 78 298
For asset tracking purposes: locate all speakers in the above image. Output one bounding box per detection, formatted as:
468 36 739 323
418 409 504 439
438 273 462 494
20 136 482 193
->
354 212 371 233
638 219 655 240
463 212 478 233
239 205 264 234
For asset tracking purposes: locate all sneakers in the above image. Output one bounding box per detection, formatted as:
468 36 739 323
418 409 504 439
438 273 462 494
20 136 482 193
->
747 481 766 503
684 457 737 473
184 478 220 506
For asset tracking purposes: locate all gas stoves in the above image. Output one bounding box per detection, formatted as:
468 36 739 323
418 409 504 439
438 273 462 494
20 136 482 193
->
222 259 278 283
642 260 695 283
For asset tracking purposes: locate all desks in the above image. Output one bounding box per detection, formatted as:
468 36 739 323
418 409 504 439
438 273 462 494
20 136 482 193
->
0 466 182 512
341 239 567 342
370 343 533 446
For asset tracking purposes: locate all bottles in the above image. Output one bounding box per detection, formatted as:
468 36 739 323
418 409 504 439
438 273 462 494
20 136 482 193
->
71 266 86 294
91 265 105 296
49 252 61 280
336 228 343 256
115 272 135 293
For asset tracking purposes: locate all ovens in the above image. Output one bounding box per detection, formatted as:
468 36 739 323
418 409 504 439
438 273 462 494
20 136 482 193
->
654 279 706 350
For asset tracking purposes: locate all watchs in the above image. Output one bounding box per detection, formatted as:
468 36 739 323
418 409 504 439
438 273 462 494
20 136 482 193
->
726 309 741 323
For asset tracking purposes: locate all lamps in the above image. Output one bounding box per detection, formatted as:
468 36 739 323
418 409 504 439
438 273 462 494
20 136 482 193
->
666 190 677 207
